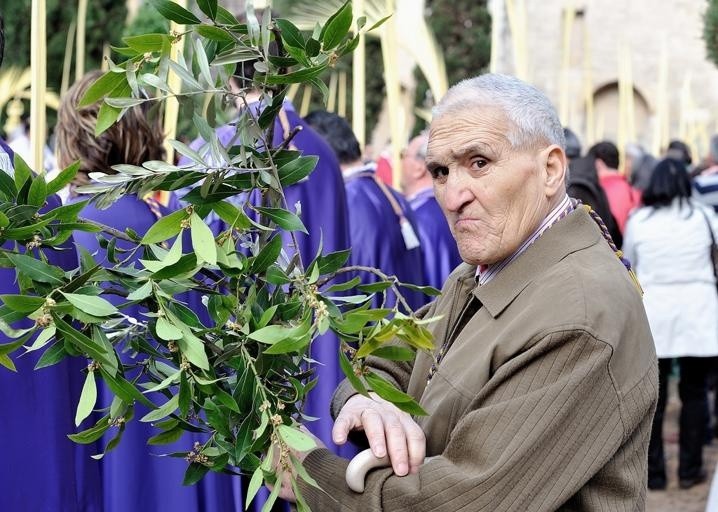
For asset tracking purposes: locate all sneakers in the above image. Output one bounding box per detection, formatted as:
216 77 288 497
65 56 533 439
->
679 470 708 489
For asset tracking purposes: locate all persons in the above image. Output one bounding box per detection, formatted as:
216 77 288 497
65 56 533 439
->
164 7 351 512
0 108 717 510
266 74 660 512
